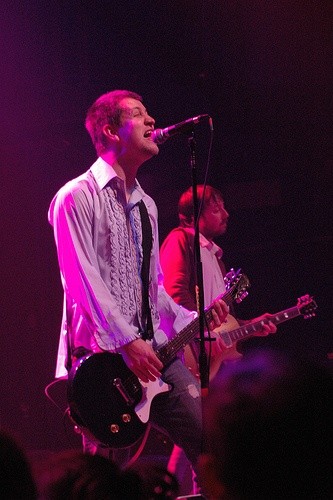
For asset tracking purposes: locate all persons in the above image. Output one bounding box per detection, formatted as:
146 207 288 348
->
47 90 230 485
0 347 333 500
158 183 276 392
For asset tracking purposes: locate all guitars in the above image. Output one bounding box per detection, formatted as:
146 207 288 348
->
69 268 251 450
182 293 318 385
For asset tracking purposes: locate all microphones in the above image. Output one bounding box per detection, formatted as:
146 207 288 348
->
151 114 209 144
